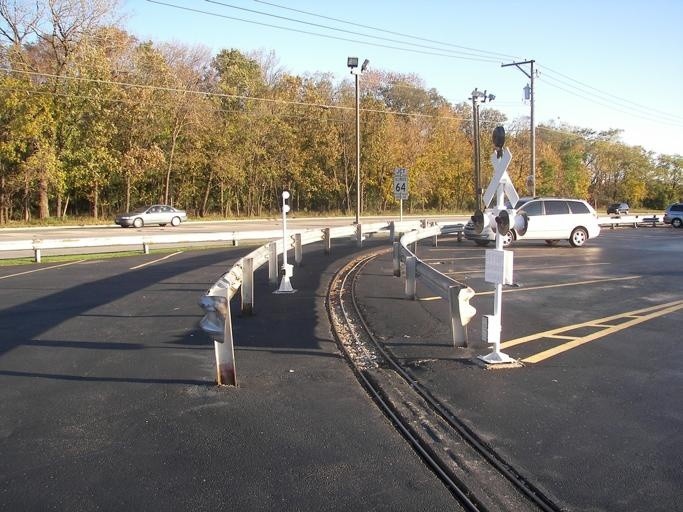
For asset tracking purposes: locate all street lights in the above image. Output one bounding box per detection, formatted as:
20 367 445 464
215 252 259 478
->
523 84 535 197
348 58 370 224
468 90 495 210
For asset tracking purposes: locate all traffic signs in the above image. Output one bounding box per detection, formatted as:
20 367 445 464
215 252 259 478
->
394 168 408 200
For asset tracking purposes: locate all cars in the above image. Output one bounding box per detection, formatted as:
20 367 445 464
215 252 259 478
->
115 205 186 228
607 203 628 214
464 197 600 248
663 203 683 228
